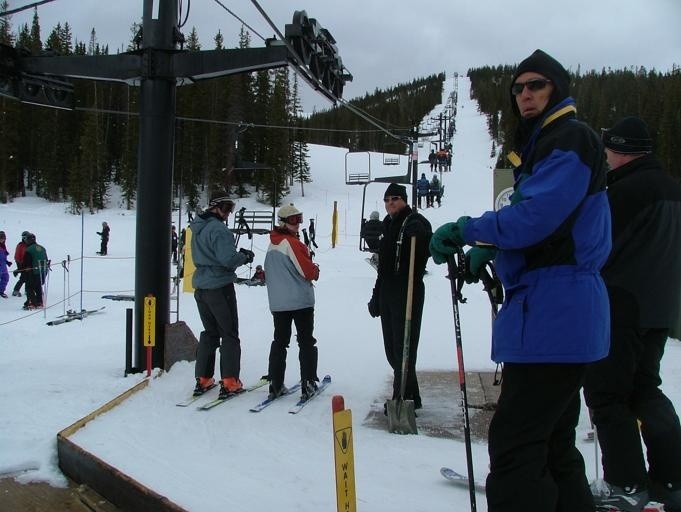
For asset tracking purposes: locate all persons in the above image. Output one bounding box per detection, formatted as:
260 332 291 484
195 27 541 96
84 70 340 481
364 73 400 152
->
428 49 614 512
252 265 265 285
12 231 30 297
583 118 681 512
182 191 254 393
362 149 452 250
172 225 177 263
264 206 319 401
23 233 48 306
368 183 432 417
96 222 110 255
0 231 12 297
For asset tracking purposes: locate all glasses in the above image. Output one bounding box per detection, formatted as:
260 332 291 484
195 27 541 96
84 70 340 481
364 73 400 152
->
278 212 302 225
384 196 402 202
210 200 236 213
510 79 551 95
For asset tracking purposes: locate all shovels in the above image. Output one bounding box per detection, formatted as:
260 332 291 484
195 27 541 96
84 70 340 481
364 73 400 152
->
387 237 418 434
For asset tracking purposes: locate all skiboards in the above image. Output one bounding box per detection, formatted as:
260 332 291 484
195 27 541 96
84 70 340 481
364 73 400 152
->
176 375 331 413
46 307 106 325
442 467 486 491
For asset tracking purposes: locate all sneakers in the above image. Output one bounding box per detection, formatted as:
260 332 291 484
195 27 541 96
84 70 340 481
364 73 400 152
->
268 381 285 394
200 377 215 389
0 294 7 297
591 479 681 512
13 291 21 296
302 381 317 396
24 299 43 306
222 378 242 392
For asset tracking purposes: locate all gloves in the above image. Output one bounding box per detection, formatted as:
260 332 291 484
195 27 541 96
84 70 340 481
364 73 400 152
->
240 248 254 265
96 232 100 234
7 261 12 267
14 271 20 277
462 246 496 284
406 220 428 241
429 215 473 265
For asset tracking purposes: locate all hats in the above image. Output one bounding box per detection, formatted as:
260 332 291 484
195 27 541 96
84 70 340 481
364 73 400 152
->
102 222 106 227
209 190 233 211
602 119 652 154
422 173 425 178
508 50 571 122
385 183 407 204
278 208 300 226
22 231 29 238
0 231 6 240
26 234 36 243
433 174 437 179
370 211 379 220
